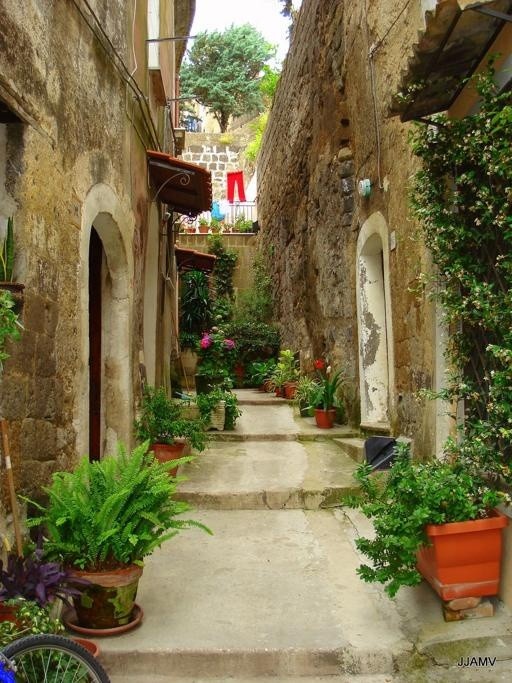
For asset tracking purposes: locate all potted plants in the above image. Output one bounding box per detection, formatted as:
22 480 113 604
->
219 321 316 418
0 383 242 683
0 212 24 321
176 215 254 234
337 440 507 604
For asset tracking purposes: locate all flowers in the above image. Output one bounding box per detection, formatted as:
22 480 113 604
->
192 330 239 374
312 359 347 410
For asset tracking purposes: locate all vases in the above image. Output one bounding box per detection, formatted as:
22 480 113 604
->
194 372 224 395
314 408 337 429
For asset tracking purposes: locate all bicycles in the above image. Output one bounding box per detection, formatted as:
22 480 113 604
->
0 631 112 683
